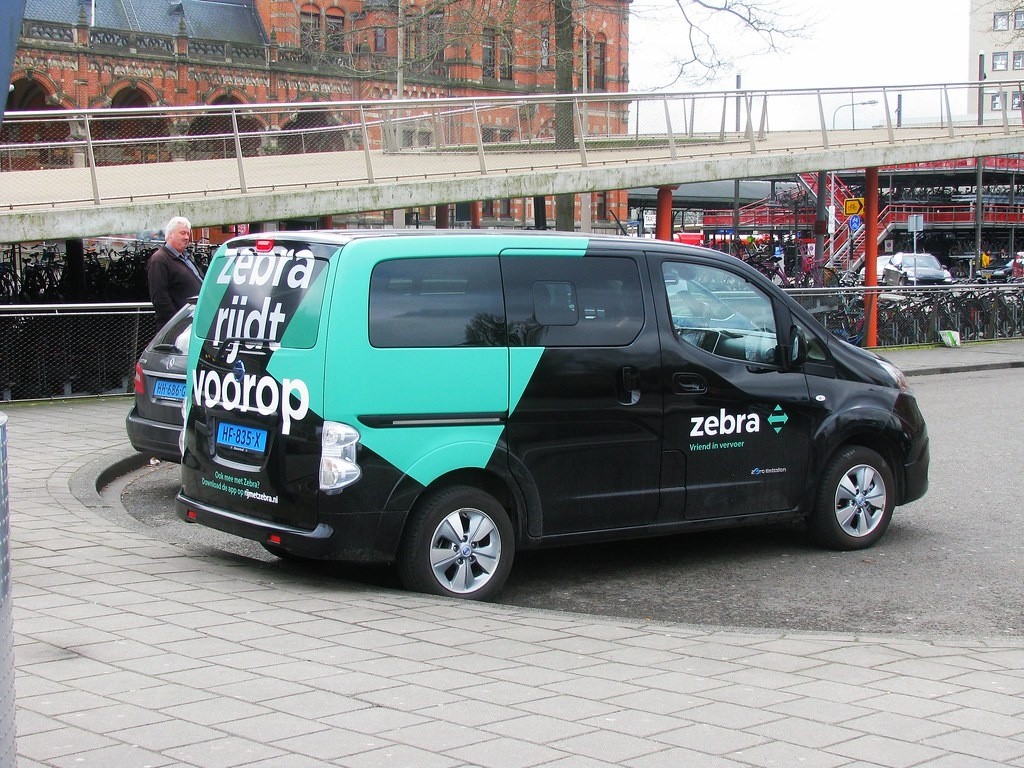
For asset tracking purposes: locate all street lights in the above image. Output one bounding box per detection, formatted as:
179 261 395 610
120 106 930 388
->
832 100 879 129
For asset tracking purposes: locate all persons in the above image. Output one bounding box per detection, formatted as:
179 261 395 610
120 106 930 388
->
982 250 992 281
997 249 1008 260
146 216 204 333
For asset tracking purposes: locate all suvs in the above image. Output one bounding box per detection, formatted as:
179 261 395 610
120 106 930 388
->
882 252 953 289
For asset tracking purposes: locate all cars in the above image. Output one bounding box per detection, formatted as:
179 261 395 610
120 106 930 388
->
975 257 1015 284
663 276 761 330
859 254 894 285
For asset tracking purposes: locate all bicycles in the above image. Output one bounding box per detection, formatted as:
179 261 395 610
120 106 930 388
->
768 178 1024 213
0 237 222 309
694 224 1024 347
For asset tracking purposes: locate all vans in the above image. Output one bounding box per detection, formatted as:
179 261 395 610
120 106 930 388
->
125 294 199 465
173 226 931 599
